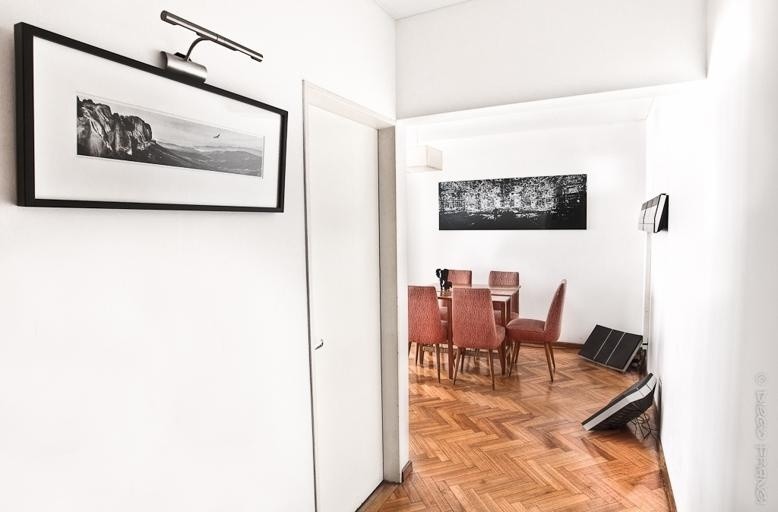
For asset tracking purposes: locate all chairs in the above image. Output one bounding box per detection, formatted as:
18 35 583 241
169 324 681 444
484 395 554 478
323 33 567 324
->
407 270 568 391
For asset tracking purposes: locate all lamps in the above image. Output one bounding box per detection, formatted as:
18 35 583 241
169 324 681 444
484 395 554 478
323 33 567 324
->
406 133 443 175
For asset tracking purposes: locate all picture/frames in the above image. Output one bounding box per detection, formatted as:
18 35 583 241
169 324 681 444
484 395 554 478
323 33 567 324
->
14 21 288 213
